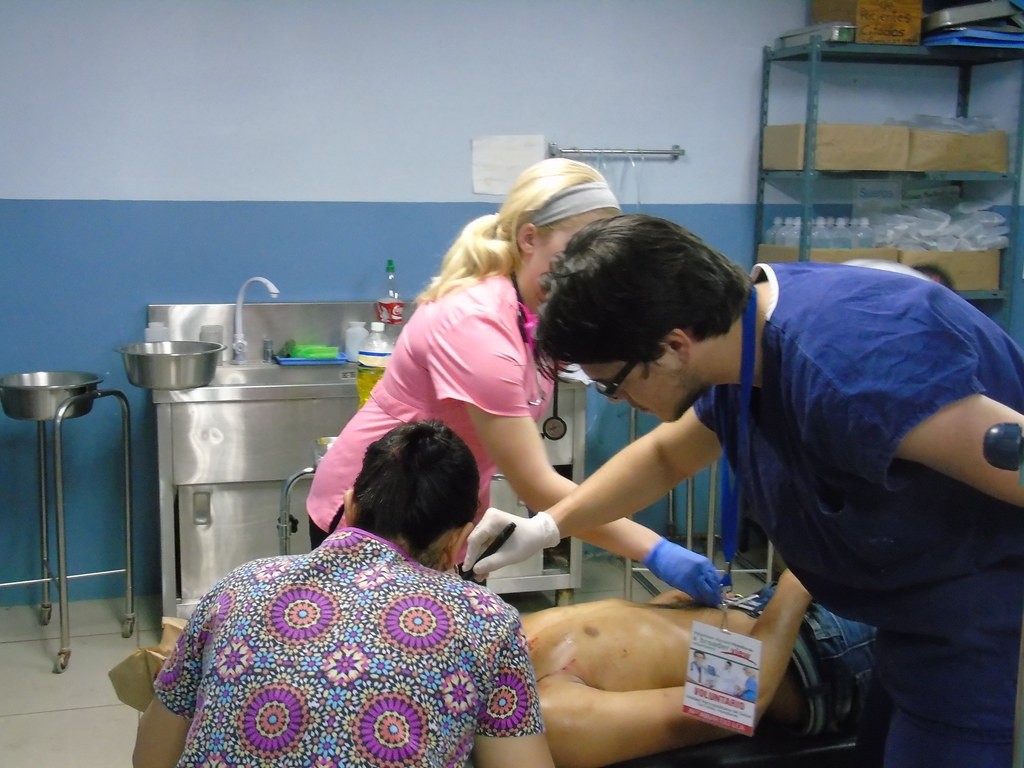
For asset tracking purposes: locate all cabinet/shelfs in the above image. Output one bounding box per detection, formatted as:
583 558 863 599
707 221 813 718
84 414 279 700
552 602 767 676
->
730 35 1024 585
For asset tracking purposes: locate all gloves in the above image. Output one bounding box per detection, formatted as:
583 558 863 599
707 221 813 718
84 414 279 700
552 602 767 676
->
641 536 721 607
462 507 560 583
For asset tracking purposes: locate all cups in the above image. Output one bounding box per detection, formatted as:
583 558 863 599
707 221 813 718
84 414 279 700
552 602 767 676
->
202 325 223 364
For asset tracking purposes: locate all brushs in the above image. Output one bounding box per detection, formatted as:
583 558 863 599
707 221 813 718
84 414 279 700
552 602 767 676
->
289 342 340 358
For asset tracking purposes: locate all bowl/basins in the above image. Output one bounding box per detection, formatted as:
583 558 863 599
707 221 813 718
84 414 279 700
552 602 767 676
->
114 341 229 390
0 371 104 421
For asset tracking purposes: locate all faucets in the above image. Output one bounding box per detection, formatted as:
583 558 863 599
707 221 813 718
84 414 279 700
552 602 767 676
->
233 275 280 365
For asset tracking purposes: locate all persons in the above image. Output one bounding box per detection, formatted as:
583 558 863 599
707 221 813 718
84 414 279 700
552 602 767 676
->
133 418 555 767
304 157 1024 768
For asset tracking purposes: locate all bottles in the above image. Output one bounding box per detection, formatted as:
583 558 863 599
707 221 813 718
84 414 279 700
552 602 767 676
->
765 216 875 248
144 322 167 341
377 260 404 345
357 322 395 410
345 321 369 362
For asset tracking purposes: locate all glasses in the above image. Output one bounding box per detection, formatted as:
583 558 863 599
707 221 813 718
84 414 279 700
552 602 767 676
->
595 361 637 398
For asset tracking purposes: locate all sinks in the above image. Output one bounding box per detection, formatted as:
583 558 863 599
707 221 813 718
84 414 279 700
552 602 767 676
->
149 357 368 481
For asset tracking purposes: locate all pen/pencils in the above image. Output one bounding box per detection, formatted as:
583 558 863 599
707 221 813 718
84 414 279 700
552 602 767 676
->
462 521 516 584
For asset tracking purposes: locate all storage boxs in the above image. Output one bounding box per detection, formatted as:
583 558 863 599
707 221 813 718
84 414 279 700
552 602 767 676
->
810 0 923 46
762 124 909 171
908 128 1008 174
757 243 898 263
897 249 1001 291
